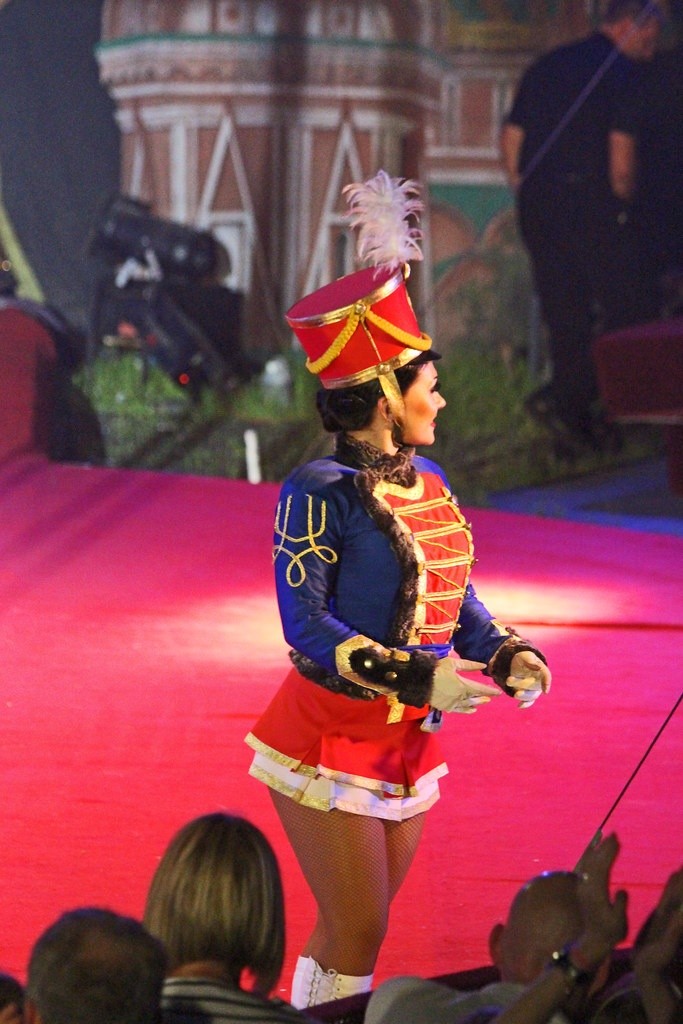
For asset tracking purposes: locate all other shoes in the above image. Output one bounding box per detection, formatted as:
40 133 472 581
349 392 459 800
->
524 381 603 453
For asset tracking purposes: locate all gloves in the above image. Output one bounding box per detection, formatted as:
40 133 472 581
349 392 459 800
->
427 657 502 714
506 650 551 708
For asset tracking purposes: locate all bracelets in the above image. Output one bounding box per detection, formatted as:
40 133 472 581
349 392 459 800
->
548 946 585 988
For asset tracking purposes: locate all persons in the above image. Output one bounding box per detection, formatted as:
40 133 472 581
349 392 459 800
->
489 0 675 455
361 869 614 1024
2 906 166 1023
454 829 683 1024
140 812 320 1023
242 261 552 1011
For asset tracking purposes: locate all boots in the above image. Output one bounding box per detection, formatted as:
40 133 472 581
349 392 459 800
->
294 957 374 1008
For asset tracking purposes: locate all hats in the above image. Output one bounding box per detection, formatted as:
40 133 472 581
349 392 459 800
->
285 169 443 389
